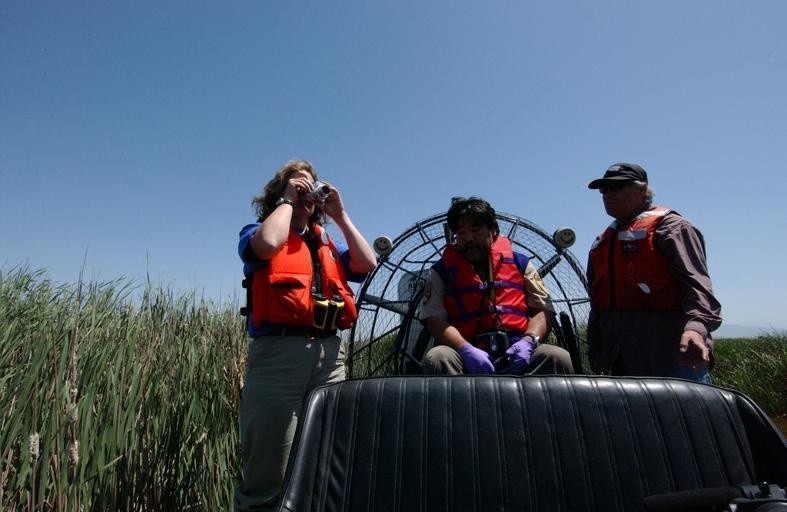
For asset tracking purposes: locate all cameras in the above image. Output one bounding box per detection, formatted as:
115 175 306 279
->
305 180 332 207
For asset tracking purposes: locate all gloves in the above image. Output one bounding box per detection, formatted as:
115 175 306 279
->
501 337 538 375
458 342 496 374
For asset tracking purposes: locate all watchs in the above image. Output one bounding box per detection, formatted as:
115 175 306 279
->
275 198 295 207
523 332 542 347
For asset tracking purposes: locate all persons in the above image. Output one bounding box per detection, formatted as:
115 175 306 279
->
233 158 379 511
583 160 724 384
418 194 575 378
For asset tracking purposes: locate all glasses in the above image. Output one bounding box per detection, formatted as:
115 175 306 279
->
598 181 626 192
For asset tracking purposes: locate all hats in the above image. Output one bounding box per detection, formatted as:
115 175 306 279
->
588 162 648 189
